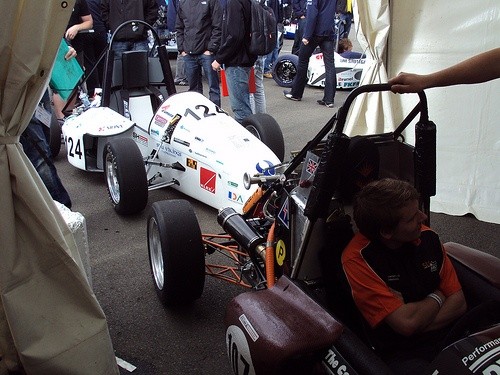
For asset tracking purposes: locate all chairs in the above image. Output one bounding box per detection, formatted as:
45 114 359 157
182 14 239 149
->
119 50 165 117
316 137 420 337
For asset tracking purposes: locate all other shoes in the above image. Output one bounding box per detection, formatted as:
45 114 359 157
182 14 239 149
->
284 90 301 101
178 81 188 86
318 99 334 107
263 71 272 78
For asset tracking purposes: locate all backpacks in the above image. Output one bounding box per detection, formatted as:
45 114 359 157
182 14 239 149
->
250 0 277 57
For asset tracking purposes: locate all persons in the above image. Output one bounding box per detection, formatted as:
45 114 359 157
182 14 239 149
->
211 0 284 124
292 0 348 55
388 47 500 94
338 38 367 59
167 0 192 86
283 0 337 107
176 0 222 109
341 178 468 375
100 0 158 57
45 0 108 139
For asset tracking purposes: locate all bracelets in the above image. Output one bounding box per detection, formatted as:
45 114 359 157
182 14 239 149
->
430 293 443 308
